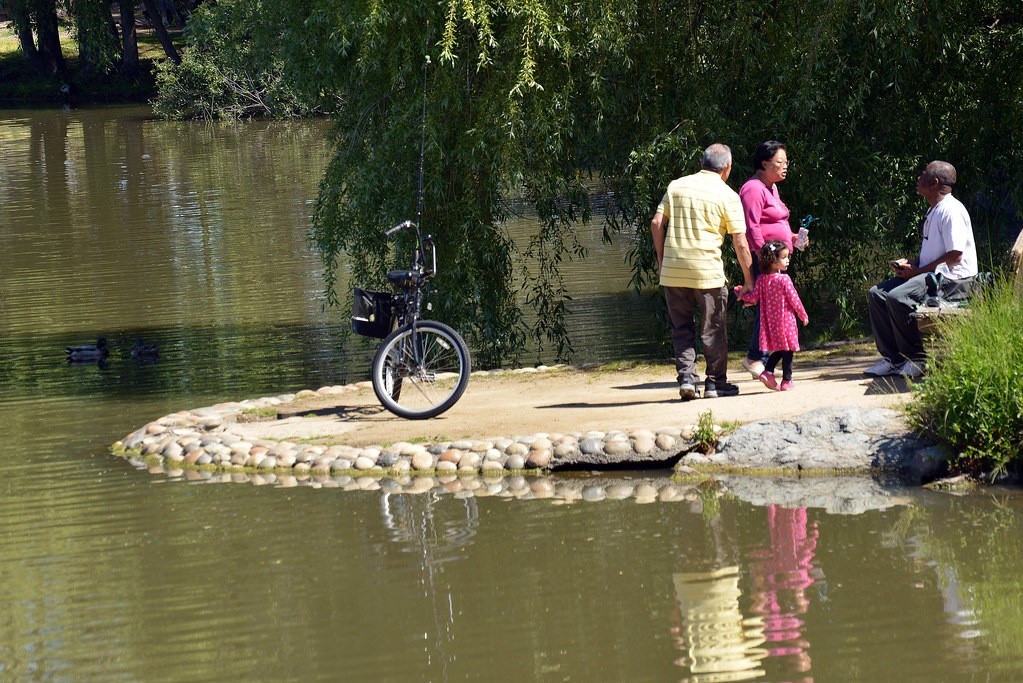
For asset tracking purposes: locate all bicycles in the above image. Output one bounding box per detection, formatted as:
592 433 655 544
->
353 222 471 420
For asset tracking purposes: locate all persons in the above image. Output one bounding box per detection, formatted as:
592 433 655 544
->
739 141 809 379
863 160 979 378
651 143 757 401
734 238 809 392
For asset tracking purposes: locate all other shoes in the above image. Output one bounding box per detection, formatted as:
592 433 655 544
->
743 358 765 377
780 380 796 390
759 370 780 390
752 368 783 380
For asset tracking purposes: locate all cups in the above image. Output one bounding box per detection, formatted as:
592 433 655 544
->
794 227 809 248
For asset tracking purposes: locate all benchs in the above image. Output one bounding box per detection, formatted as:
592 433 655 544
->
915 272 996 369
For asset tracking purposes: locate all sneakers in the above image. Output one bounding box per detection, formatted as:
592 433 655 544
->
863 356 907 377
679 375 701 401
704 378 740 397
900 361 926 379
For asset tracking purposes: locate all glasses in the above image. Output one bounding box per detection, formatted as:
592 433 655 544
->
768 159 790 167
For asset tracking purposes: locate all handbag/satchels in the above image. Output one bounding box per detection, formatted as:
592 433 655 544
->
350 288 391 340
